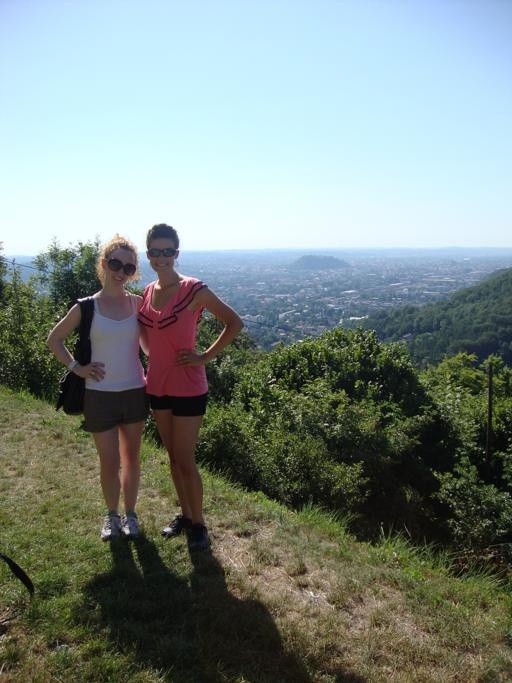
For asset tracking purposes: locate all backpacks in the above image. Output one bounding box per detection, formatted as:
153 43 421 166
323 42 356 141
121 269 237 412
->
54 295 96 416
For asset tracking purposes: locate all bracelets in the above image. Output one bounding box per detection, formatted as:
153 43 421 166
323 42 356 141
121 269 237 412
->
68 361 79 370
200 352 208 364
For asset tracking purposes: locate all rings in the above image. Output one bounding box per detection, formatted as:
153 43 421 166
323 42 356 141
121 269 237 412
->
184 356 187 359
91 371 95 375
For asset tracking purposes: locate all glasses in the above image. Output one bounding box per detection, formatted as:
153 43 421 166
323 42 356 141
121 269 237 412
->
148 247 177 258
104 257 138 277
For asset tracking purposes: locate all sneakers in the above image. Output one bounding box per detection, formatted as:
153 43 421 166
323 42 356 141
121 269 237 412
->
121 515 140 537
100 513 122 542
187 523 212 554
160 516 189 539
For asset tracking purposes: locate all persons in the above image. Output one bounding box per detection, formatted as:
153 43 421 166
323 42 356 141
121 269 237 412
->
138 222 244 550
47 235 149 542
